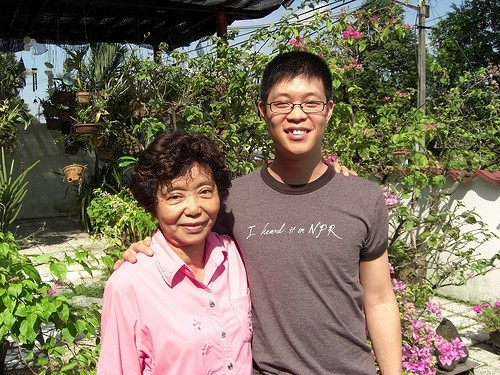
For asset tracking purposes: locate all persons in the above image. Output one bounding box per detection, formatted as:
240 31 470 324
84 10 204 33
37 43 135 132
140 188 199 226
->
113 50 402 375
95 131 253 375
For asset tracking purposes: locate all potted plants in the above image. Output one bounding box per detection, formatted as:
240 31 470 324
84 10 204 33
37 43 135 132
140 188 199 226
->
49 162 89 200
36 43 131 161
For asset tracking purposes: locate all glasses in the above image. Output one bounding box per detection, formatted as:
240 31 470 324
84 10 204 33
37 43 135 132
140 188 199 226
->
266 100 328 114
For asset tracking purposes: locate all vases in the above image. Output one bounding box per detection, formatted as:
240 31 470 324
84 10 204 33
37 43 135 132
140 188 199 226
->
490 330 500 348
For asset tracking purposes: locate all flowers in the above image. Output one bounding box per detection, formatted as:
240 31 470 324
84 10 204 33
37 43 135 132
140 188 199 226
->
471 297 500 335
